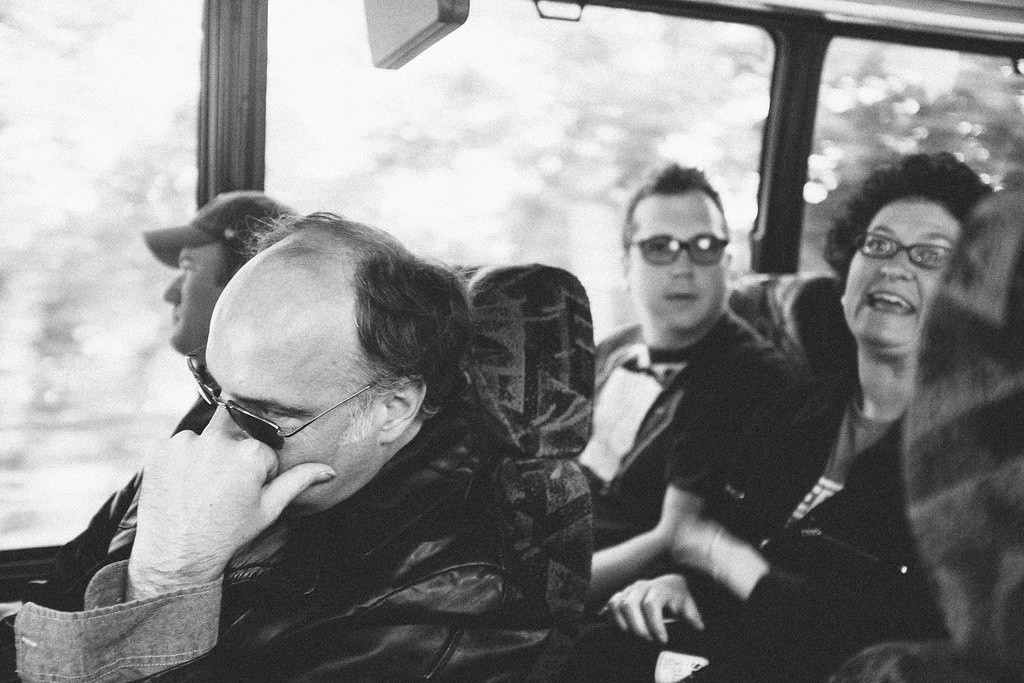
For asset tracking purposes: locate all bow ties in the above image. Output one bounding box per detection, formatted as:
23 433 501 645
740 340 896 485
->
622 348 676 387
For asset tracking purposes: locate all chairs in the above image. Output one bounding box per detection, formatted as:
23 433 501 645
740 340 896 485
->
445 261 594 621
725 272 857 387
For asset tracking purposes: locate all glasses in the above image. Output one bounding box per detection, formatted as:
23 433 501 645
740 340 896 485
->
187 342 399 451
626 232 729 266
854 233 955 270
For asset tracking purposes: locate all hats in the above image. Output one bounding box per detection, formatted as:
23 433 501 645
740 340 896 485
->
143 190 301 269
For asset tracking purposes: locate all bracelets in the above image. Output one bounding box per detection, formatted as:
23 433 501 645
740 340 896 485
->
707 525 727 580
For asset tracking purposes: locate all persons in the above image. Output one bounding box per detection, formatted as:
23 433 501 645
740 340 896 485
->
828 183 1024 683
572 162 810 609
8 213 554 683
561 145 1000 683
0 184 299 683
